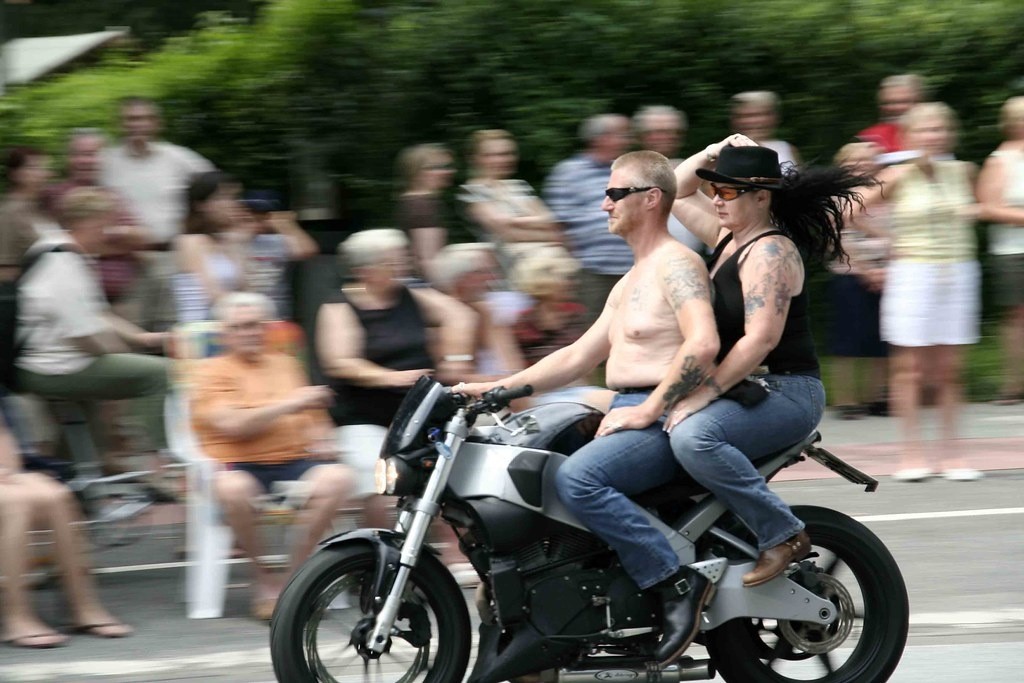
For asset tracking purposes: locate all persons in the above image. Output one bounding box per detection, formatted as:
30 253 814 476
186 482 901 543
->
0 186 203 647
317 129 582 585
830 75 983 480
663 133 888 585
733 92 803 177
0 95 214 325
544 114 634 296
450 150 721 666
976 96 1023 406
172 170 318 326
634 105 705 257
192 293 354 621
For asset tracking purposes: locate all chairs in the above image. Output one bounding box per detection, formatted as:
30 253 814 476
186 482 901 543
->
163 322 390 620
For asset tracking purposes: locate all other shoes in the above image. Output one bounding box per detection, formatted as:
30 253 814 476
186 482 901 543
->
947 468 983 482
895 467 930 479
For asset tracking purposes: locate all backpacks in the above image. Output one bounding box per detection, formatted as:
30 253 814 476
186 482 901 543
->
0 244 84 396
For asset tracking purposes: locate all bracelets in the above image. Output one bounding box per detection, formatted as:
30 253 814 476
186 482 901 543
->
442 355 474 361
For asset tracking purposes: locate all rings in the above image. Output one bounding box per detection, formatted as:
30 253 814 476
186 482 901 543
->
606 424 610 428
674 411 678 414
615 424 622 430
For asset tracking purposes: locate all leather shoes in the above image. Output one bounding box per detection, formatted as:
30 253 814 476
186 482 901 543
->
645 564 716 669
742 528 812 586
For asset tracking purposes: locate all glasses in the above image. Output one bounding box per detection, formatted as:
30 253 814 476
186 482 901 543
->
606 186 664 201
710 182 766 200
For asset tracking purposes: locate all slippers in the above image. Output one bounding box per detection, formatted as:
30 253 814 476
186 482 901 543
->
66 620 134 639
1 626 67 648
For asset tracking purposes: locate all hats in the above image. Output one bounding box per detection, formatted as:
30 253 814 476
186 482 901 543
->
696 146 785 183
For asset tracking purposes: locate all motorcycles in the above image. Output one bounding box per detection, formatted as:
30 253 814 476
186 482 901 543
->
265 369 910 683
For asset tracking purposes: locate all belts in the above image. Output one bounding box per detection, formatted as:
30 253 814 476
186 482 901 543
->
619 386 659 393
